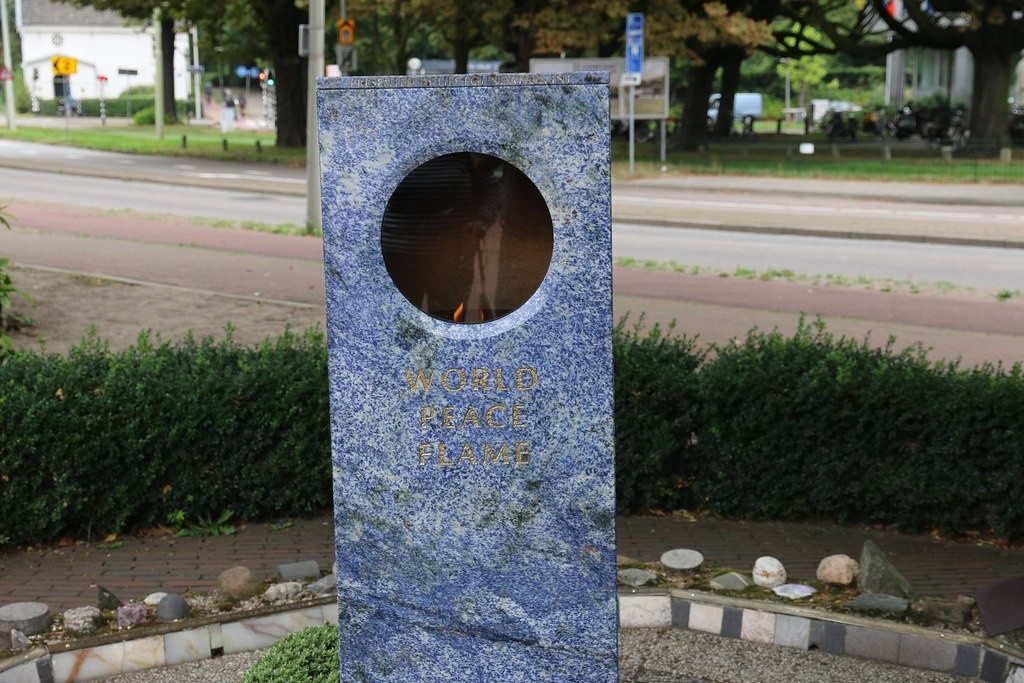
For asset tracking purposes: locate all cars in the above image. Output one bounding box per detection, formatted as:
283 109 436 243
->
225 93 246 108
893 106 951 144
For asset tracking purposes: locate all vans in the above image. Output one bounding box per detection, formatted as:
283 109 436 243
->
704 92 764 128
